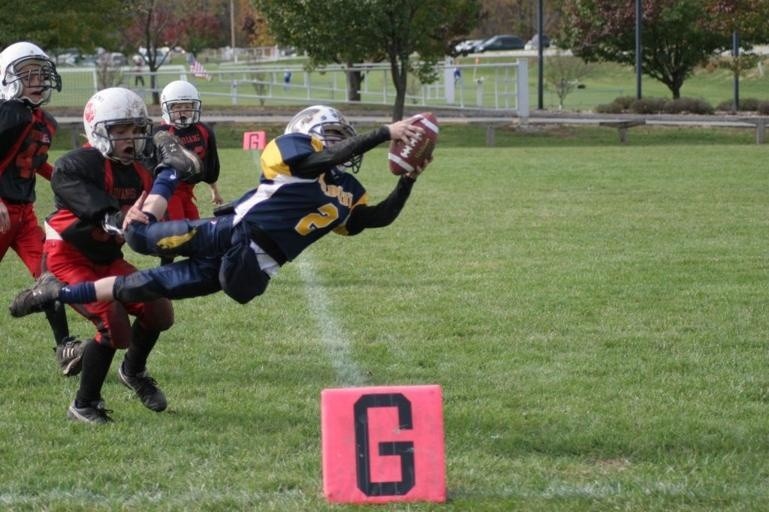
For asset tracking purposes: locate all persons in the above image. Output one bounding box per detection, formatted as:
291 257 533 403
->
135 56 145 87
144 79 224 266
0 40 92 381
454 66 461 82
7 105 434 317
283 67 291 89
46 84 175 427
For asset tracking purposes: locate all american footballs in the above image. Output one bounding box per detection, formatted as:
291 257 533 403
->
389 112 439 174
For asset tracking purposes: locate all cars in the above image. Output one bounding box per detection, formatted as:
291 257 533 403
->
451 31 558 58
42 43 187 67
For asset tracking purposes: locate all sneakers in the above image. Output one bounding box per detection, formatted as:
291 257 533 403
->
67 400 115 425
9 272 63 318
118 361 168 412
56 337 91 375
153 130 208 185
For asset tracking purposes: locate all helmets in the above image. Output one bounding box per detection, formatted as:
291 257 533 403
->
160 80 201 133
0 41 63 107
83 87 155 163
284 105 364 174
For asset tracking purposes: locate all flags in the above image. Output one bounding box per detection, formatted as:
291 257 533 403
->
186 54 212 80
